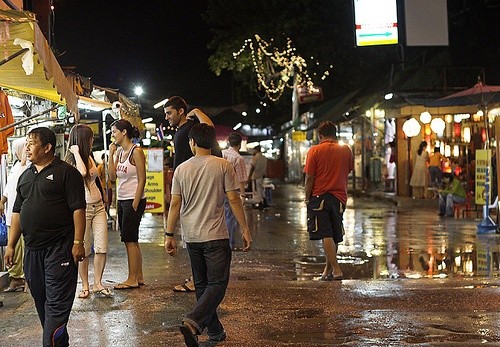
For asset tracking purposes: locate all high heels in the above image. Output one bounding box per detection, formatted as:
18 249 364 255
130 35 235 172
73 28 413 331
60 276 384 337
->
4 280 28 293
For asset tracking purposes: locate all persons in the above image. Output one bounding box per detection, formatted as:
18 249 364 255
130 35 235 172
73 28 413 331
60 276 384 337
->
0 137 33 292
385 137 447 198
4 127 87 347
436 172 467 218
303 121 353 281
97 153 114 218
164 123 255 347
186 115 222 160
63 123 116 299
247 144 269 212
108 119 147 291
163 96 216 294
219 132 248 250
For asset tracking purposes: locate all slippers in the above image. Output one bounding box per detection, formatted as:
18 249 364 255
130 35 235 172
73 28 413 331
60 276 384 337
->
206 336 227 342
114 282 145 290
180 325 200 347
231 246 243 251
173 278 195 292
319 272 343 280
78 289 90 298
93 288 113 298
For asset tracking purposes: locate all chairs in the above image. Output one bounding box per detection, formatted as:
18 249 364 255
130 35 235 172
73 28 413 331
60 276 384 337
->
454 191 474 218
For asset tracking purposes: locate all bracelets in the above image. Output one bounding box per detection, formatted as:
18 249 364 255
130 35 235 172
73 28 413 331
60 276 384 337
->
165 231 174 237
73 240 86 245
304 199 309 203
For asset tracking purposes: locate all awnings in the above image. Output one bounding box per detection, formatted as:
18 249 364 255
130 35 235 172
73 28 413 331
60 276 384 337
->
1 9 80 138
77 84 149 135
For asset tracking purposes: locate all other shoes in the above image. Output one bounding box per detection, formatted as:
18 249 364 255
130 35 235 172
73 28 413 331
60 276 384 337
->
420 196 425 199
253 205 272 212
437 214 453 217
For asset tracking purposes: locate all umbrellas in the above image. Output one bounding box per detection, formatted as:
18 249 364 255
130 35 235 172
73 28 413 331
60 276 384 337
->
430 77 500 146
